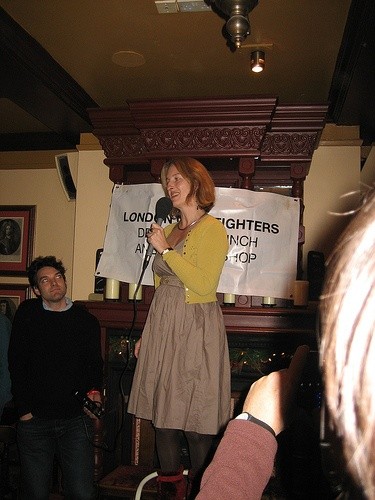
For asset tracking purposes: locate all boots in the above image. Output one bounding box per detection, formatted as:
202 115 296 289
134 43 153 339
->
156 464 188 500
189 465 207 500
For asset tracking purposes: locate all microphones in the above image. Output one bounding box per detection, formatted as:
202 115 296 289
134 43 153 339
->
143 197 173 269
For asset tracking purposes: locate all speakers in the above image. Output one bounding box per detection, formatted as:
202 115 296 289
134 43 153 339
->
55 152 78 200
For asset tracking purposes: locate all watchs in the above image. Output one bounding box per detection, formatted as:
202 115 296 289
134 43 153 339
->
235 413 275 436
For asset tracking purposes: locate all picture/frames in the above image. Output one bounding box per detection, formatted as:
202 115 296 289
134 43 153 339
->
0 283 32 317
0 204 37 277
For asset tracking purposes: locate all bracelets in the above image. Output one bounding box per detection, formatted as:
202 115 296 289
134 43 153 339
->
161 248 173 255
87 390 100 394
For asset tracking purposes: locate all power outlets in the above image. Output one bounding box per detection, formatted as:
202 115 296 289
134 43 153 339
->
177 0 212 12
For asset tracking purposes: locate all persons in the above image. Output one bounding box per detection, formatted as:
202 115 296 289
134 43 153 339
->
134 157 231 500
195 180 375 500
0 311 13 415
9 255 103 500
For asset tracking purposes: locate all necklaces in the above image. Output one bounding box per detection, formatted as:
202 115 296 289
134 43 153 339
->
178 213 207 230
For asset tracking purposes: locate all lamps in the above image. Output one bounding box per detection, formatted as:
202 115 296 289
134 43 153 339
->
250 51 265 73
